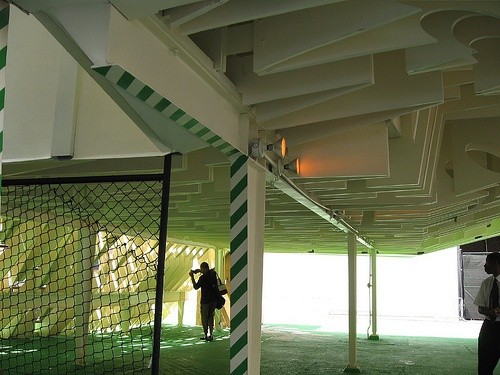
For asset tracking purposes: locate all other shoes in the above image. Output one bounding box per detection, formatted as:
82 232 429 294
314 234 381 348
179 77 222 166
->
200 337 208 340
207 335 214 341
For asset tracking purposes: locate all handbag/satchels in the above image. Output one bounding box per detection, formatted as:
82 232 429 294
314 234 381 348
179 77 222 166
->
215 296 226 310
215 272 229 296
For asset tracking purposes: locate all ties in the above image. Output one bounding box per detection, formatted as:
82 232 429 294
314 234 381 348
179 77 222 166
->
489 279 500 321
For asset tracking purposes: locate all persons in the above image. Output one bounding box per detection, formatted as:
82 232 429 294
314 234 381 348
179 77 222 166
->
473 252 500 375
189 262 228 340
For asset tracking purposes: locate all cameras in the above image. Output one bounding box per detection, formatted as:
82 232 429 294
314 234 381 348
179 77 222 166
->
191 270 196 274
